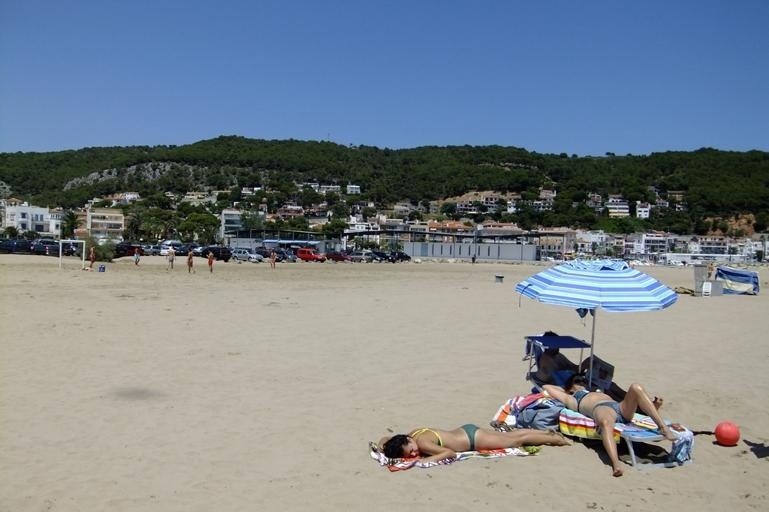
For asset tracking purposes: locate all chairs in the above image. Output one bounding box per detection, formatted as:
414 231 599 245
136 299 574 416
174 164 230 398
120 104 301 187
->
494 335 694 466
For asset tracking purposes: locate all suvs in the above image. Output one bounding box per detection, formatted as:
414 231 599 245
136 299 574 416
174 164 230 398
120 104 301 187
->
297 248 327 263
231 249 263 262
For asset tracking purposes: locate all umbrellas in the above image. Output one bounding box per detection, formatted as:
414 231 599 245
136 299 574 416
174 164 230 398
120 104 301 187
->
515 256 680 388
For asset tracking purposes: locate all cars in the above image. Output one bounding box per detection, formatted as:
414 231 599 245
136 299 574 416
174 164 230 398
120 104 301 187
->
1 236 78 258
115 238 232 262
326 248 411 263
255 246 297 263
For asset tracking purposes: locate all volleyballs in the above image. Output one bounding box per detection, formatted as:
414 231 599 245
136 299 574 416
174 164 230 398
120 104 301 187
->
715 421 740 447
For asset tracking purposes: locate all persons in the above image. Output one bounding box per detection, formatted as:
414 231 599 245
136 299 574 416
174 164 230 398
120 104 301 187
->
376 423 577 466
165 247 177 270
271 249 278 267
90 244 96 268
187 248 196 273
205 249 214 272
542 373 678 476
708 263 714 277
534 332 663 416
134 246 143 265
